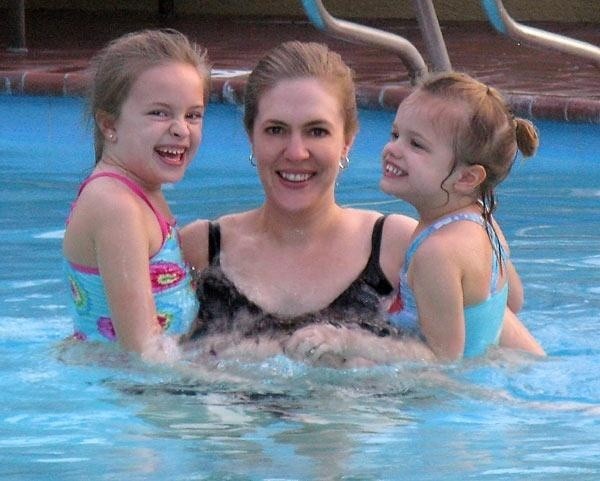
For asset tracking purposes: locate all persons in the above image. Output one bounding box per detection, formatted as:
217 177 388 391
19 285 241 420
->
179 41 546 370
62 27 214 351
284 70 538 367
